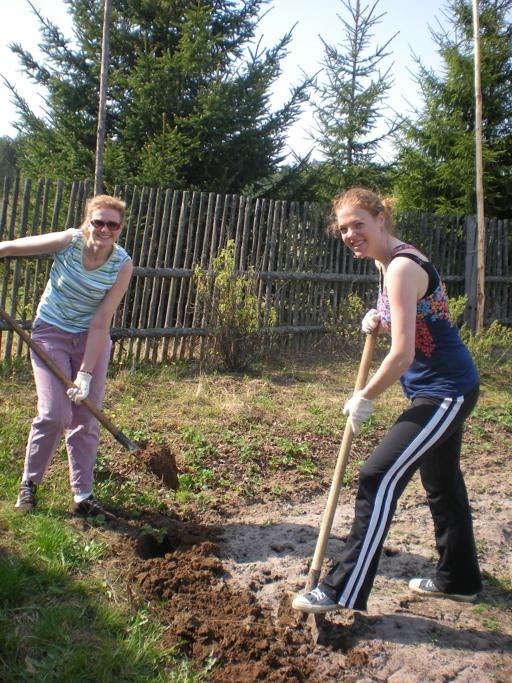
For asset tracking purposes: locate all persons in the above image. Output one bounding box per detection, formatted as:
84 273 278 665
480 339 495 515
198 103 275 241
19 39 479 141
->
292 189 483 615
0 194 134 518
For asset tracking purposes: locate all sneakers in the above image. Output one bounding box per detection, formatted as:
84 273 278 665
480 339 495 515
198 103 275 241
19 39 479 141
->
15 481 37 513
409 579 476 602
292 588 344 613
71 494 116 520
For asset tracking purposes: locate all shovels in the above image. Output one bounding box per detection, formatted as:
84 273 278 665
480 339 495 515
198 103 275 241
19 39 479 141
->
0 304 178 489
276 318 381 644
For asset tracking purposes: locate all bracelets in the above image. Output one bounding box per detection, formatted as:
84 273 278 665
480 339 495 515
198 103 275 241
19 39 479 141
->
77 370 92 375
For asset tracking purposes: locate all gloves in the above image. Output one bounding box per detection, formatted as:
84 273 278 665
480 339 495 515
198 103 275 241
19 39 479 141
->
66 372 92 405
362 309 389 335
342 391 373 436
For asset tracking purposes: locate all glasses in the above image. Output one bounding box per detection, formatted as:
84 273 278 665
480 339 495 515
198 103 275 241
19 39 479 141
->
91 220 123 230
